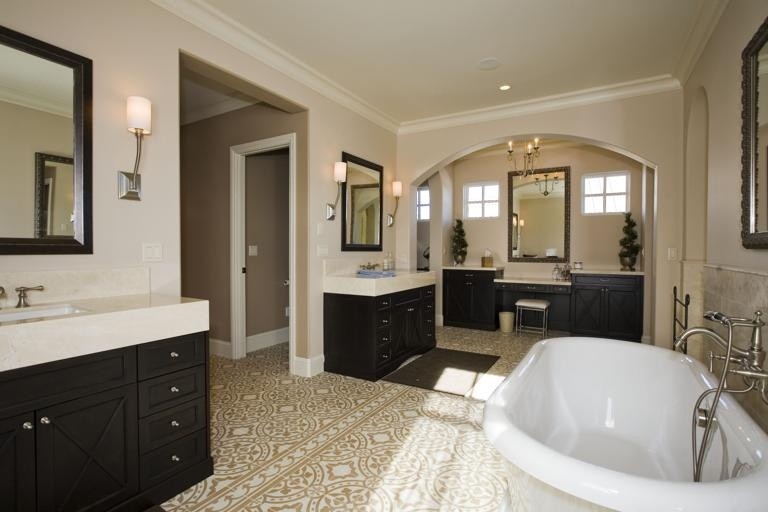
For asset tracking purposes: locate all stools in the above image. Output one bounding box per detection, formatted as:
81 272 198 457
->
512 299 550 339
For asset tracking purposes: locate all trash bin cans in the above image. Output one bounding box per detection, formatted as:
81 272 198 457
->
499 312 515 332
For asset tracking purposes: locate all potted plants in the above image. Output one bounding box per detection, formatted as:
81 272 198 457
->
451 218 468 265
618 212 642 271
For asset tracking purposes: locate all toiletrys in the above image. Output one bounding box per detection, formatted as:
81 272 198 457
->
383 252 389 270
552 260 571 280
389 251 394 269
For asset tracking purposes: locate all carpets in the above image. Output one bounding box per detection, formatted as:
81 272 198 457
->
382 345 501 397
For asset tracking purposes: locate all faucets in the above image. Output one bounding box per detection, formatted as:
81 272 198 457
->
0 283 6 298
673 324 750 366
367 261 380 269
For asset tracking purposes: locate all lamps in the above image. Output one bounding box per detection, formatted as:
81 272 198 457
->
386 181 403 226
505 134 541 178
534 172 558 196
326 160 348 219
118 94 153 199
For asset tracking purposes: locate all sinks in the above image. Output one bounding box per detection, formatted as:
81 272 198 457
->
356 268 396 278
0 303 88 322
483 335 762 510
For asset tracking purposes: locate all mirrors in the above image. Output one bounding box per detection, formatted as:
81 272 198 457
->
341 152 384 251
34 153 75 240
507 168 571 264
739 18 768 249
0 26 94 256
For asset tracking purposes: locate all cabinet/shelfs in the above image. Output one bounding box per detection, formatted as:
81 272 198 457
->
492 283 572 293
571 275 643 343
0 330 210 512
324 285 435 381
442 270 502 332
